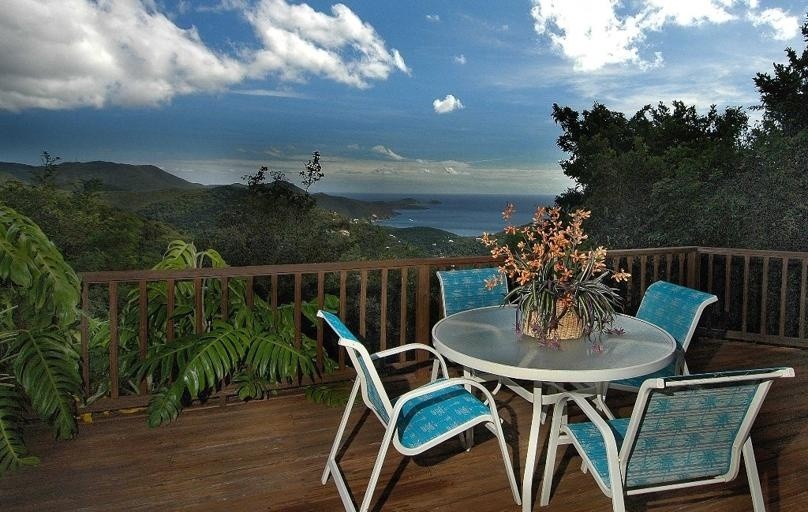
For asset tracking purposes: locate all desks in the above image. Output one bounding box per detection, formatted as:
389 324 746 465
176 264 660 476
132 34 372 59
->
431 305 678 511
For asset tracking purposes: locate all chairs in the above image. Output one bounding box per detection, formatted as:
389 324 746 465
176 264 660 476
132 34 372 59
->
431 267 553 453
540 366 795 512
541 280 718 475
318 308 524 511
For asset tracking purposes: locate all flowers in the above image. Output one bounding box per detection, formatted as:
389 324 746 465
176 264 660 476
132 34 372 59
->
475 201 632 351
